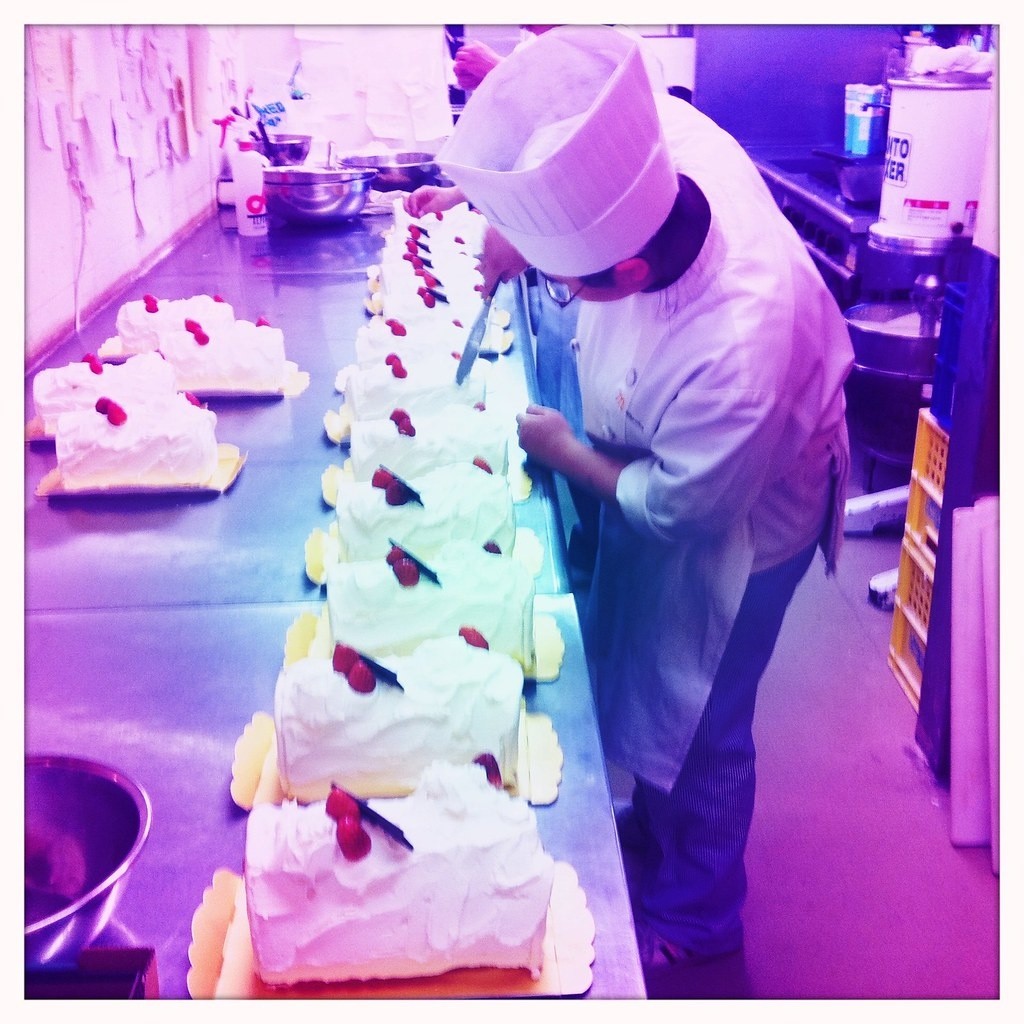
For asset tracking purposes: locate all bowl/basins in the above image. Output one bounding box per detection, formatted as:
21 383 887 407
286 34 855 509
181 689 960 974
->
24 753 150 972
339 152 440 190
262 173 377 225
258 134 311 164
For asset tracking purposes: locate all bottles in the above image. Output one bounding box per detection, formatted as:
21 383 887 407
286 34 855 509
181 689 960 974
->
232 141 268 237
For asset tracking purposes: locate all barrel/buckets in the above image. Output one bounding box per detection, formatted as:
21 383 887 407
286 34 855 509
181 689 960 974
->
881 79 995 226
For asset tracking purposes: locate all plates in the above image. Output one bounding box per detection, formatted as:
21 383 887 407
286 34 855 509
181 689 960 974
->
263 166 377 184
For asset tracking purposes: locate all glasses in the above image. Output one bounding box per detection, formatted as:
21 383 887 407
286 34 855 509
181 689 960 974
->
540 273 586 307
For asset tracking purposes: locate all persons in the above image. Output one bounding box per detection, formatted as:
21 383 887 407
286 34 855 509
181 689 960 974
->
433 24 856 970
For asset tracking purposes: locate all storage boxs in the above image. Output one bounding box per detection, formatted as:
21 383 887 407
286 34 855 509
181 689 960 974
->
888 282 968 716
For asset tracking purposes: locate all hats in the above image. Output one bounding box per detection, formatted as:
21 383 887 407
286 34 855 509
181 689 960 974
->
433 26 679 277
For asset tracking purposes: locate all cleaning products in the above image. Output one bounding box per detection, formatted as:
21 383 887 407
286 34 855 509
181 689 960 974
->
213 116 268 239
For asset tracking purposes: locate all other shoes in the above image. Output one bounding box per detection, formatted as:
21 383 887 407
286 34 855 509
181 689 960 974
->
612 796 653 853
635 913 736 977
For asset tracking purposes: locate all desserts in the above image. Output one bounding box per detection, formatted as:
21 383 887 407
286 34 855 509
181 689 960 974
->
32 197 559 985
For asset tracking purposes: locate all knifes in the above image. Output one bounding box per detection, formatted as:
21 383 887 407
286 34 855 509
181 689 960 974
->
454 276 501 384
